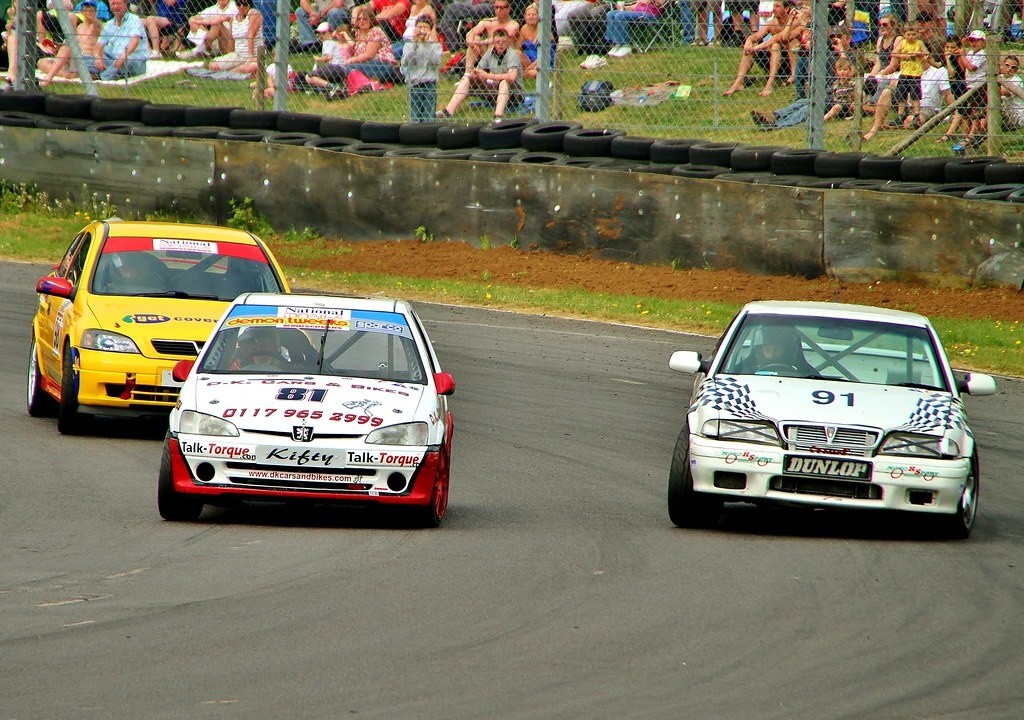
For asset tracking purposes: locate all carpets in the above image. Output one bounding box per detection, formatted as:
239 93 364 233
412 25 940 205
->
0 59 204 85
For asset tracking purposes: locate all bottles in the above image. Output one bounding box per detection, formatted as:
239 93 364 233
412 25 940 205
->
312 61 319 72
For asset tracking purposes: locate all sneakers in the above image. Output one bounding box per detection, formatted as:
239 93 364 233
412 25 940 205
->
583 55 607 70
614 45 631 58
608 44 622 56
579 54 594 68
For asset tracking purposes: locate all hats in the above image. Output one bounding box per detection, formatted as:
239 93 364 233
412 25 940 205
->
314 22 332 35
966 30 986 41
914 10 933 22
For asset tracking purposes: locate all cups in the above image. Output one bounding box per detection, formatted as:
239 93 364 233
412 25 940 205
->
617 1 625 11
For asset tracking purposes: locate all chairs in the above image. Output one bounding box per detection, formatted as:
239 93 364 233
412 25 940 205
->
224 259 264 298
630 4 674 54
229 328 334 371
885 364 922 386
735 329 820 377
100 251 172 290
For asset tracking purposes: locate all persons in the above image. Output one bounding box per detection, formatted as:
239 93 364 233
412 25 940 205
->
5 0 1024 145
109 251 143 284
753 321 798 371
232 326 280 370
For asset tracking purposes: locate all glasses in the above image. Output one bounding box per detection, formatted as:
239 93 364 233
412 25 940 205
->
236 2 249 6
879 22 892 28
1004 62 1018 69
494 5 510 9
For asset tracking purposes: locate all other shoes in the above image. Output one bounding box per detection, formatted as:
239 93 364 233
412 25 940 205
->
911 113 921 129
889 114 907 127
750 109 779 132
493 115 504 124
935 128 987 148
435 108 453 119
326 84 339 98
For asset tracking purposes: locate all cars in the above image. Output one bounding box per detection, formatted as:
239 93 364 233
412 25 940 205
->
667 300 997 540
26 219 293 437
156 293 455 529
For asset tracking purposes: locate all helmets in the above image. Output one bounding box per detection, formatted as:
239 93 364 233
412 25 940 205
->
237 326 289 370
109 251 143 285
754 323 798 370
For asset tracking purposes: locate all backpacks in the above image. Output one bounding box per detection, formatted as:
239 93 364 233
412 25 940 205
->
577 80 614 112
